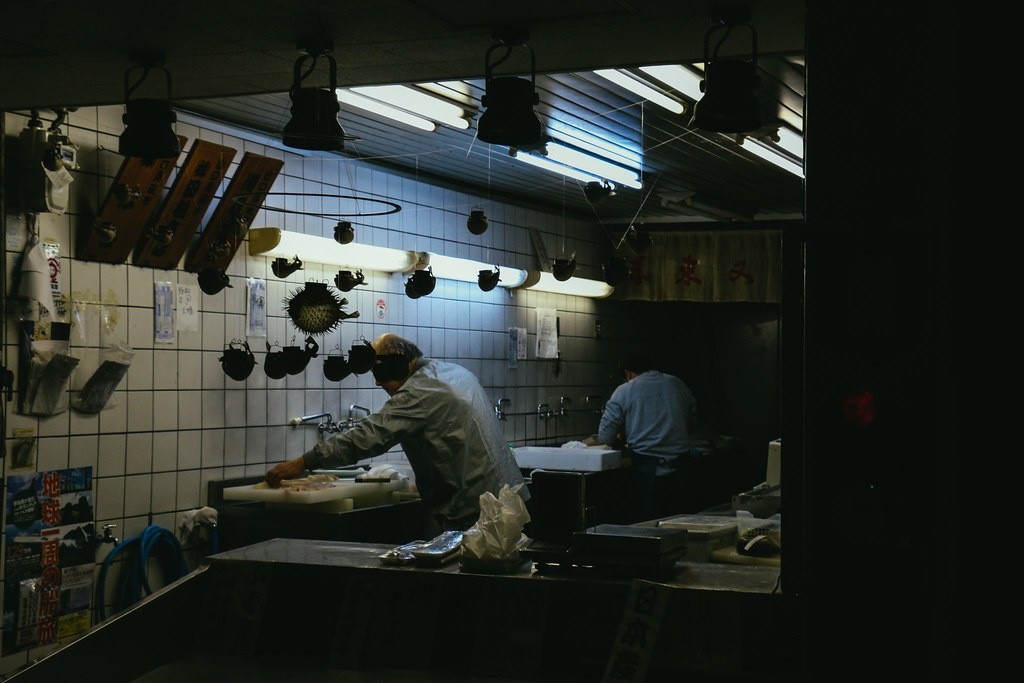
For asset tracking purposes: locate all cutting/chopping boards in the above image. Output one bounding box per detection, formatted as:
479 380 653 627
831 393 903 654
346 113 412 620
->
221 478 408 504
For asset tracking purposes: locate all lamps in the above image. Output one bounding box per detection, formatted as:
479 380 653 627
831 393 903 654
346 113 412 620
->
250 228 419 272
693 0 781 139
282 31 348 151
415 250 528 288
119 49 179 155
522 272 615 298
477 28 545 142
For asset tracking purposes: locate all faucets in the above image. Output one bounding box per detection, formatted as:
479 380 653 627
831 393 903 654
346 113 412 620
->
493 404 503 420
96 524 122 550
344 418 355 432
552 409 560 417
744 534 782 597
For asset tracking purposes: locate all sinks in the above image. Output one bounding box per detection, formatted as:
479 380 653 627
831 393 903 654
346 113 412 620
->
334 463 372 473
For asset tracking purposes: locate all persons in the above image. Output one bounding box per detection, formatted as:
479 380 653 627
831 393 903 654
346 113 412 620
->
264 333 532 541
598 350 699 519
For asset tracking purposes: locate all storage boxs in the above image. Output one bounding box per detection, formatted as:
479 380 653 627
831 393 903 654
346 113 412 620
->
511 442 622 471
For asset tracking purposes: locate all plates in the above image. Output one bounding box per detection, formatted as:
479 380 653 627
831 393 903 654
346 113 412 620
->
378 531 465 566
